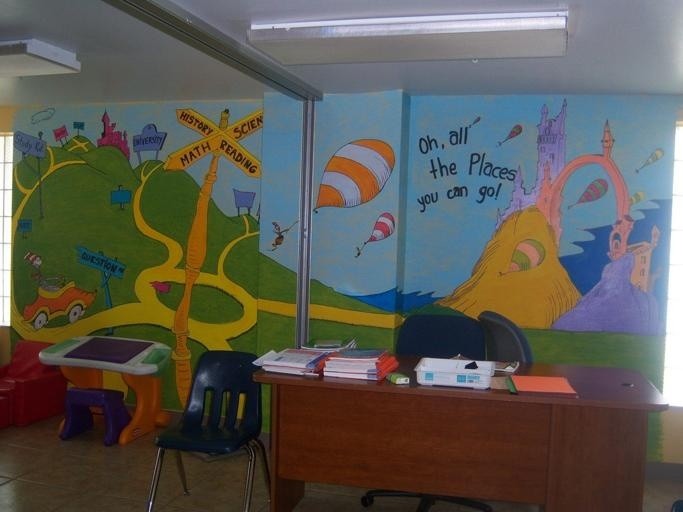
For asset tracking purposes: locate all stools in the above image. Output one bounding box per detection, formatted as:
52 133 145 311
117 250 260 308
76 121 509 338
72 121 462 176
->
61 386 132 445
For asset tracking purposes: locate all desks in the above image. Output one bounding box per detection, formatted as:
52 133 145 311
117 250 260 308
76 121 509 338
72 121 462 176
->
251 336 669 509
39 334 169 442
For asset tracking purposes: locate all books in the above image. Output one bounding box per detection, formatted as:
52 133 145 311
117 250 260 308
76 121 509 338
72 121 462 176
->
250 335 400 382
507 373 578 399
493 359 519 373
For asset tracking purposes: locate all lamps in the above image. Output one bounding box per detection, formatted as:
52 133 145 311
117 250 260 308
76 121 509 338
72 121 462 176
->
0 39 80 81
246 11 567 40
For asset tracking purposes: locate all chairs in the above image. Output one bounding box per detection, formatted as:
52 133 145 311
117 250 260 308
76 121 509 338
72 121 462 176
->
147 352 270 511
360 312 496 511
